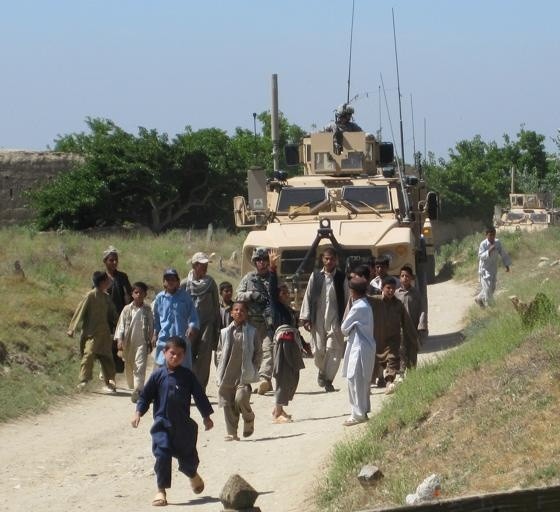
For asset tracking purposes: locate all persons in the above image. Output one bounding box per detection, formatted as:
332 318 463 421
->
475 224 510 310
132 337 214 506
65 244 427 442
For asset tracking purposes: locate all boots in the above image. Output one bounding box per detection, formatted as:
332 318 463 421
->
258 376 269 395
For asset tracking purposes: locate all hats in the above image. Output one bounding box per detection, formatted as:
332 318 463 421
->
163 269 177 277
191 252 213 263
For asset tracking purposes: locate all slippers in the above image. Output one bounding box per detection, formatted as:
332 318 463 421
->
343 417 369 425
272 410 293 424
152 493 167 506
224 418 253 442
190 473 204 495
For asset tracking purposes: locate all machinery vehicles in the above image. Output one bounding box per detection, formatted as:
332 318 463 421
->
233 3 440 351
492 164 559 240
395 92 438 287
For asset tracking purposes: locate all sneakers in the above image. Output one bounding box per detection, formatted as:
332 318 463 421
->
318 370 335 392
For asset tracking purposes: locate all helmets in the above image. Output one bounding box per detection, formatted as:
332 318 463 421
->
251 246 269 267
